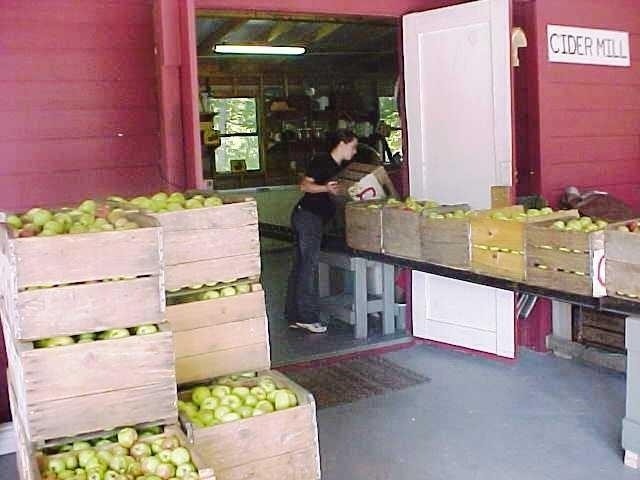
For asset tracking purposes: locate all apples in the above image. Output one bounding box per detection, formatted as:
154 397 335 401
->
6 191 223 237
28 275 136 292
177 371 297 430
36 325 157 348
356 199 640 298
165 277 250 306
37 419 198 480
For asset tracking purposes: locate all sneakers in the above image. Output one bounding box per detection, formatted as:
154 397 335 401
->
288 322 328 333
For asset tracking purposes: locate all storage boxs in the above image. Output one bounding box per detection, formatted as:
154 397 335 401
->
469 207 578 282
344 198 406 254
3 367 217 479
524 217 637 296
419 203 524 269
603 220 640 303
0 200 168 339
160 286 271 380
97 192 262 293
0 307 182 440
177 370 322 480
383 198 465 260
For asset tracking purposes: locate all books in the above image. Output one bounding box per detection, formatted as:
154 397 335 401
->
200 122 213 131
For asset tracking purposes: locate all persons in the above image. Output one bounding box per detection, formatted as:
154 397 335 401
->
286 128 359 334
